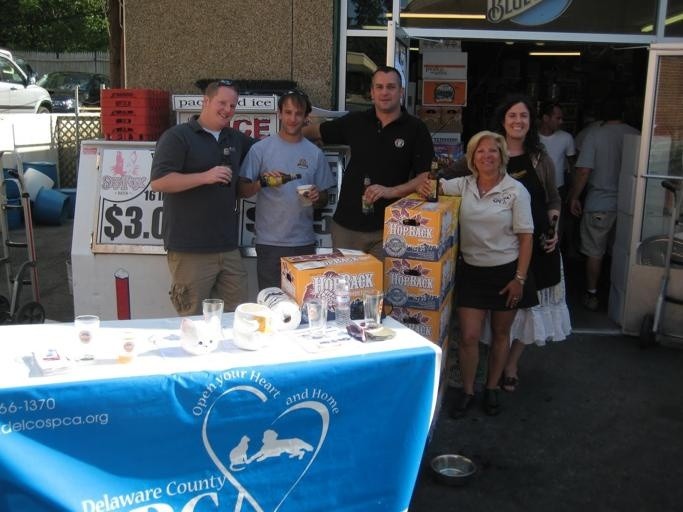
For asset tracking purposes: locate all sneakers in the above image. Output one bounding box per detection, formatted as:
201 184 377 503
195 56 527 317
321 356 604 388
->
584 292 601 311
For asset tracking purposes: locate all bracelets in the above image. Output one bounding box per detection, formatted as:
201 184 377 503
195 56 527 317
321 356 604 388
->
516 274 526 279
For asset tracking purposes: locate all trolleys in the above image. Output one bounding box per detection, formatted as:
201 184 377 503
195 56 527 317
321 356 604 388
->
640 177 683 348
0 150 45 325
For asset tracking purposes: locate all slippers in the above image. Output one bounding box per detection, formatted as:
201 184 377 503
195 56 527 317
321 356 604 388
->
502 377 521 396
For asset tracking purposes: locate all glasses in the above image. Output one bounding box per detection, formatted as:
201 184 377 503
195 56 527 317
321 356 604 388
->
217 78 236 88
284 90 307 98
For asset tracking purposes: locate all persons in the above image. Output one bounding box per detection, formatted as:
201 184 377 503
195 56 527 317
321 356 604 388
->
576 115 599 136
150 80 269 317
258 66 435 264
437 95 572 392
574 120 607 151
417 131 540 418
570 93 642 311
238 89 337 292
536 100 577 257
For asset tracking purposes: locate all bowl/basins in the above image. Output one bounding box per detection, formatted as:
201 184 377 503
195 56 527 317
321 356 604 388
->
430 454 478 486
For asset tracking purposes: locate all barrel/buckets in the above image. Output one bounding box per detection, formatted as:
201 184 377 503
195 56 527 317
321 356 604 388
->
7 198 24 231
59 188 76 218
22 161 59 189
32 185 70 226
23 167 55 203
3 167 20 200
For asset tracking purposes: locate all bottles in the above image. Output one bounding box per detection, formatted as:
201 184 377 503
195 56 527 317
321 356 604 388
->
259 172 301 188
426 157 439 203
539 215 558 253
361 167 376 217
334 275 351 328
219 143 232 187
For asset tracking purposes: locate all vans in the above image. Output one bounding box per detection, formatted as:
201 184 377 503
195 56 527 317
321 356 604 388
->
0 49 52 113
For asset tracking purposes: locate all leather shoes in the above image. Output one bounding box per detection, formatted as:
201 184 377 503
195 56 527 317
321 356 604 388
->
483 388 503 415
450 390 475 418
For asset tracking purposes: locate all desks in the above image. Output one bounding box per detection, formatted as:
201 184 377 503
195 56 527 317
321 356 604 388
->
0 310 445 507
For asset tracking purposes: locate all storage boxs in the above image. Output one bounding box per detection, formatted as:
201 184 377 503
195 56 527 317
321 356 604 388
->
383 240 459 312
422 81 467 106
384 284 454 349
613 209 672 247
609 287 626 327
618 173 670 217
99 88 170 141
383 192 462 262
611 241 631 292
424 52 467 81
621 134 672 179
279 247 384 324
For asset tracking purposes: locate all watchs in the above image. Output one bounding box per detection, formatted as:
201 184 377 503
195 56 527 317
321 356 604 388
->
515 277 525 284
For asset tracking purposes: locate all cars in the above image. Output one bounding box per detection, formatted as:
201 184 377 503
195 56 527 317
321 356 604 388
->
38 71 112 113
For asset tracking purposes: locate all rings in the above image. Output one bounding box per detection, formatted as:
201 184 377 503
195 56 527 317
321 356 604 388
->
514 297 518 301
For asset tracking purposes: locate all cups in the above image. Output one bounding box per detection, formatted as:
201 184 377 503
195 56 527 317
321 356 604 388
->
73 315 99 361
306 298 328 339
202 297 225 326
363 289 384 329
296 185 313 207
